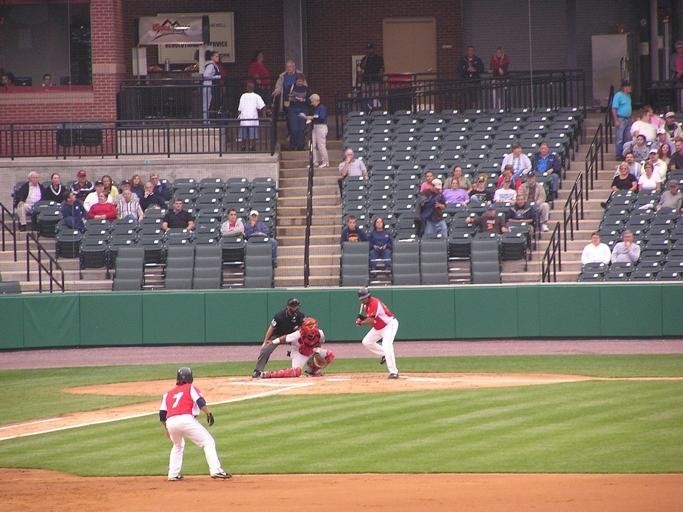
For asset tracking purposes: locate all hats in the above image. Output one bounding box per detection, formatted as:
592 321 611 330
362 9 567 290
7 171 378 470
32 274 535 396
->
249 210 260 215
649 111 679 186
621 80 632 86
431 168 536 211
75 169 132 189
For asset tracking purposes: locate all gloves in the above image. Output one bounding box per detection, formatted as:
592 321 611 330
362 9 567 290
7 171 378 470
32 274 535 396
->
207 412 214 426
355 318 362 325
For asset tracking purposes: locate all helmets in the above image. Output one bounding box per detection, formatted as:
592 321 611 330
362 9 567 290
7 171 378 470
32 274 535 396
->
286 298 301 318
302 317 317 339
178 367 194 384
358 287 371 300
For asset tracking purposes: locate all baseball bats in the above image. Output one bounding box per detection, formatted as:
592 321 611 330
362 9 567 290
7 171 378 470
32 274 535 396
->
359 304 363 314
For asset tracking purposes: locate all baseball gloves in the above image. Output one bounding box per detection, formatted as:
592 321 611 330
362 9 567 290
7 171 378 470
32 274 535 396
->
314 354 327 369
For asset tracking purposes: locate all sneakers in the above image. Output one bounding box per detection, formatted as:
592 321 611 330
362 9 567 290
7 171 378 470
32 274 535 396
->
305 368 325 376
251 368 266 378
380 354 398 379
211 471 231 479
169 474 184 480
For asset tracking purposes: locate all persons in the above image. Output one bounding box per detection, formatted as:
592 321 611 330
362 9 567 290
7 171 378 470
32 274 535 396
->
354 286 399 380
580 229 611 265
668 39 682 112
13 171 171 231
456 44 485 107
339 214 366 246
368 215 393 277
601 108 682 211
0 71 15 88
251 298 305 378
490 48 509 109
159 368 230 480
417 142 561 239
252 318 334 380
611 230 640 264
199 48 327 169
244 210 278 269
220 209 244 270
162 199 195 234
611 80 633 161
40 73 54 87
336 148 370 199
184 50 199 73
141 54 162 119
359 42 386 110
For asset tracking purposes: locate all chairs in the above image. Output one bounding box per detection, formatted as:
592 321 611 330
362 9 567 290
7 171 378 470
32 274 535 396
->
578 167 682 284
338 108 585 285
1 174 278 295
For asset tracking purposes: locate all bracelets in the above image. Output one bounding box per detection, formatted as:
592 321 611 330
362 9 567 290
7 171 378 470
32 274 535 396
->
271 337 280 345
